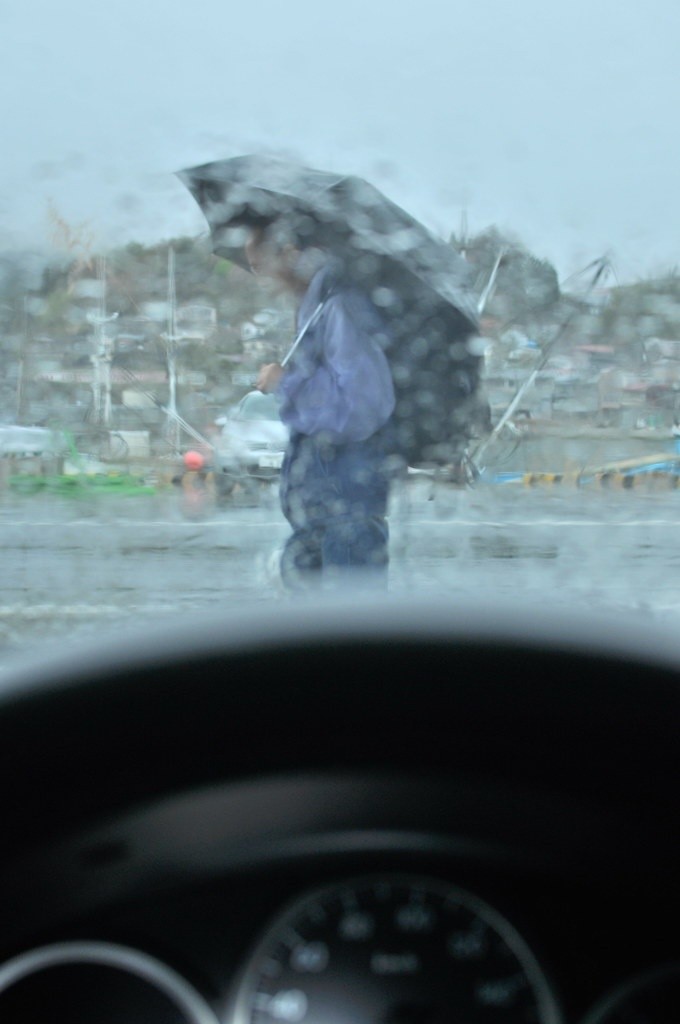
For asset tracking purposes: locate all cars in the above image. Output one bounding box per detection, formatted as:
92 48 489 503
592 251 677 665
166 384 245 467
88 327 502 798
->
213 390 289 495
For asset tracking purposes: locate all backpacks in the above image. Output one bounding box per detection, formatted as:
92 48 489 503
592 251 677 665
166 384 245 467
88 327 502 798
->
323 272 486 469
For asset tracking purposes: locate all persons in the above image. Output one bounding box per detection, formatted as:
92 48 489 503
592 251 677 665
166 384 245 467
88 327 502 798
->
173 452 216 519
243 225 396 596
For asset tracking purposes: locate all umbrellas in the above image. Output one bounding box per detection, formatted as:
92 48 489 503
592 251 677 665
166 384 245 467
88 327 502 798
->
173 153 493 469
